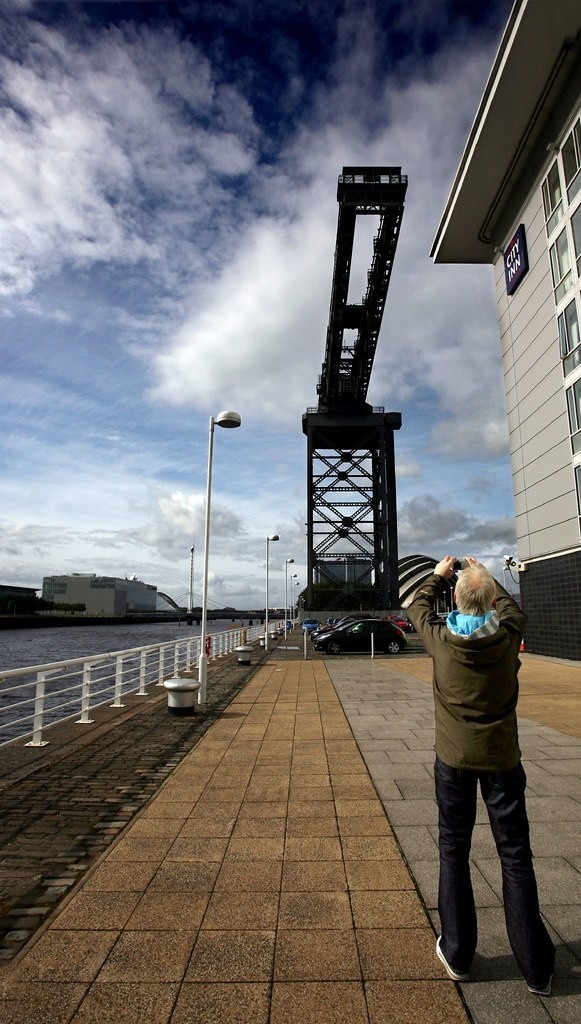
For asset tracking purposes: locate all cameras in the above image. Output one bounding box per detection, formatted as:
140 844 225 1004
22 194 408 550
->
452 560 470 570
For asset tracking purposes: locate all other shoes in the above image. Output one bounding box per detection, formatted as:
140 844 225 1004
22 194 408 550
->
528 972 553 995
436 935 469 980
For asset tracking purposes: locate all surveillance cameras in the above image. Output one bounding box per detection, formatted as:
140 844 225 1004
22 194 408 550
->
504 555 513 570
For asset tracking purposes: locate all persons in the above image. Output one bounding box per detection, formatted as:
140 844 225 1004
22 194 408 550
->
407 556 557 996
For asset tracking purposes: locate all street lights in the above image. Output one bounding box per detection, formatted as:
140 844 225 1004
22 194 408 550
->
290 573 298 634
264 535 280 652
195 410 242 703
187 546 197 614
293 582 300 628
283 558 294 640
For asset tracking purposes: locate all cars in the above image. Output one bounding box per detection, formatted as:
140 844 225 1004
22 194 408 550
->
313 618 408 656
311 614 381 642
388 615 410 632
300 618 320 635
